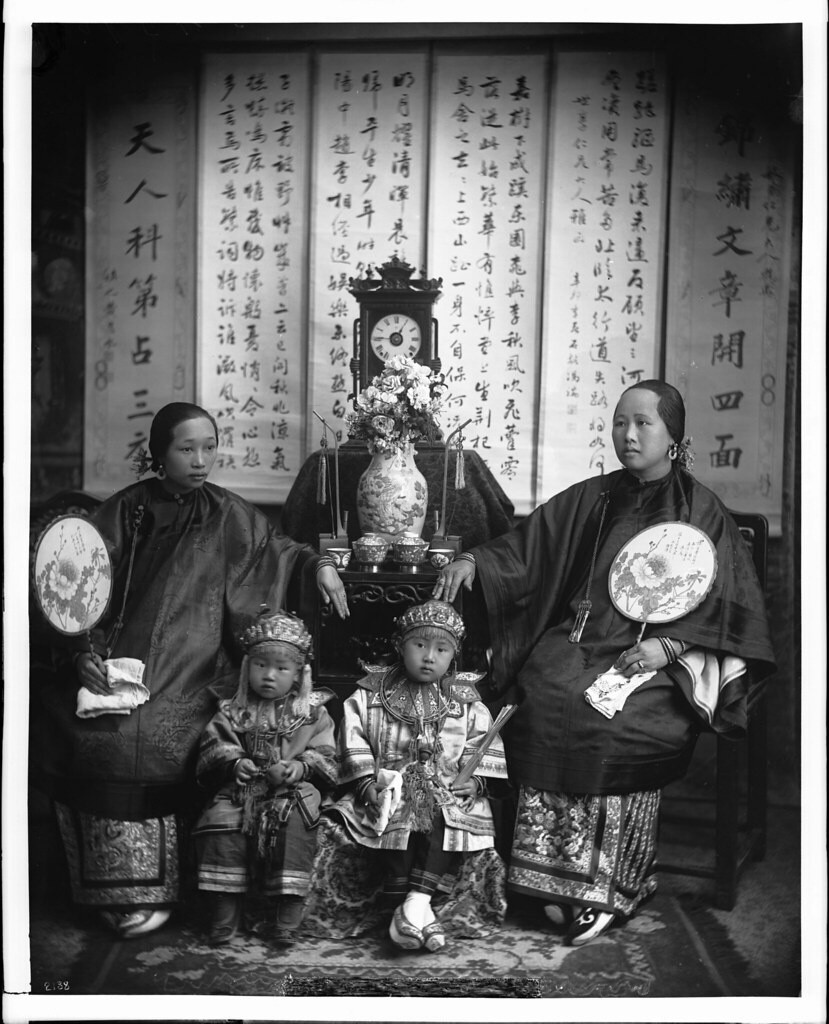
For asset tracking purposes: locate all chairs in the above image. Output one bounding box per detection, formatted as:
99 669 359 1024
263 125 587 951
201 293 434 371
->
656 509 771 911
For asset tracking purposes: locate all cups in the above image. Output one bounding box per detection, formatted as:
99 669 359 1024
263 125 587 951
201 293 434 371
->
327 532 457 571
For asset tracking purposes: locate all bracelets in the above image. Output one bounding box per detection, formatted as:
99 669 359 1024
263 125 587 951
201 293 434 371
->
315 555 337 575
232 757 243 777
659 637 686 664
453 551 477 563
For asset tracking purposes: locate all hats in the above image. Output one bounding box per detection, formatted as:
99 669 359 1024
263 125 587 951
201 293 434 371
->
234 603 313 718
394 600 464 654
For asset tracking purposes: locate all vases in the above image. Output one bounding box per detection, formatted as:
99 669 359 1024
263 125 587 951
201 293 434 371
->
356 442 428 550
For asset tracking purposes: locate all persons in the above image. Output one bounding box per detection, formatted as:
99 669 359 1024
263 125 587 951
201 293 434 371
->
340 600 508 952
190 608 338 949
31 400 350 943
431 379 776 945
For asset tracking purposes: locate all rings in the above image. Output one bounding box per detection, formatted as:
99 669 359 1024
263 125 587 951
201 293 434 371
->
638 661 644 668
469 795 475 799
364 801 370 807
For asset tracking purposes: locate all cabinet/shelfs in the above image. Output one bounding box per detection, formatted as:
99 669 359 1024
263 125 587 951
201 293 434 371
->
313 563 463 689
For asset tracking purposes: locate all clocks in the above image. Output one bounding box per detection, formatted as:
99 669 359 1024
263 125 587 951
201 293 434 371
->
339 258 446 451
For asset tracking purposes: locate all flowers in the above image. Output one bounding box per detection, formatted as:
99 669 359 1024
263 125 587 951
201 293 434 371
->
343 354 442 453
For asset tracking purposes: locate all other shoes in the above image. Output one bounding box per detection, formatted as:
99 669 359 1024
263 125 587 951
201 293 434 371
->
211 901 240 944
119 907 170 936
543 904 567 926
569 905 614 945
97 908 124 929
270 901 304 944
424 917 445 952
390 905 423 951
